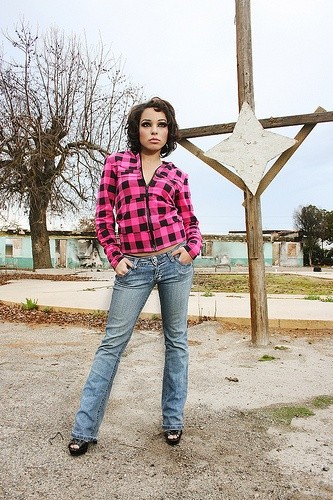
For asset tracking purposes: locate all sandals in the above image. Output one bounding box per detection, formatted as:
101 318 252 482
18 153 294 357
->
164 430 183 444
69 438 97 456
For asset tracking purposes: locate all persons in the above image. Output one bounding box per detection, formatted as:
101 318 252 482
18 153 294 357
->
67 94 203 456
